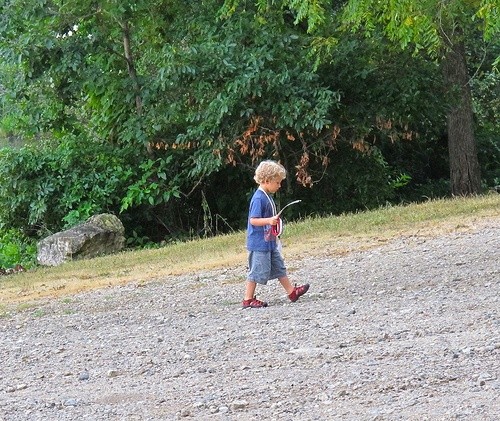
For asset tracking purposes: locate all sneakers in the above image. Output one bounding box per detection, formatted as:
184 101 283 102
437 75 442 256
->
288 283 310 303
242 295 268 307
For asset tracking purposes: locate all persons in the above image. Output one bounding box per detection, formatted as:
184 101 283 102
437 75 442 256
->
242 160 310 308
